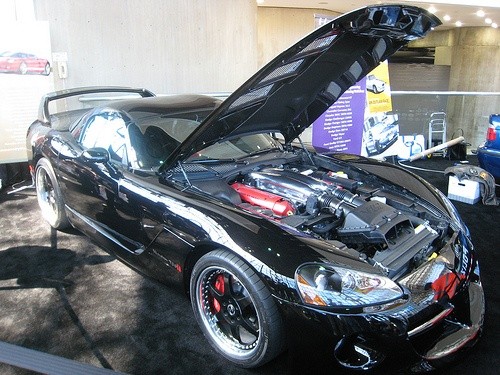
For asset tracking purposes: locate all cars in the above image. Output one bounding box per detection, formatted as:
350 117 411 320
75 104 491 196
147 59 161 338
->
363 111 398 157
25 1 485 375
0 48 51 76
366 74 387 94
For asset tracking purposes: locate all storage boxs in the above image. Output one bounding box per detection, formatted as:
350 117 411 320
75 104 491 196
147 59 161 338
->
447 176 482 205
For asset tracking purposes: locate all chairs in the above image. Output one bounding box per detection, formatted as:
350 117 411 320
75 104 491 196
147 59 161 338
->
144 126 197 164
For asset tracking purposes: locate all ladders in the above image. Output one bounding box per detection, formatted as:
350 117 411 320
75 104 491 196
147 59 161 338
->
428 112 447 157
